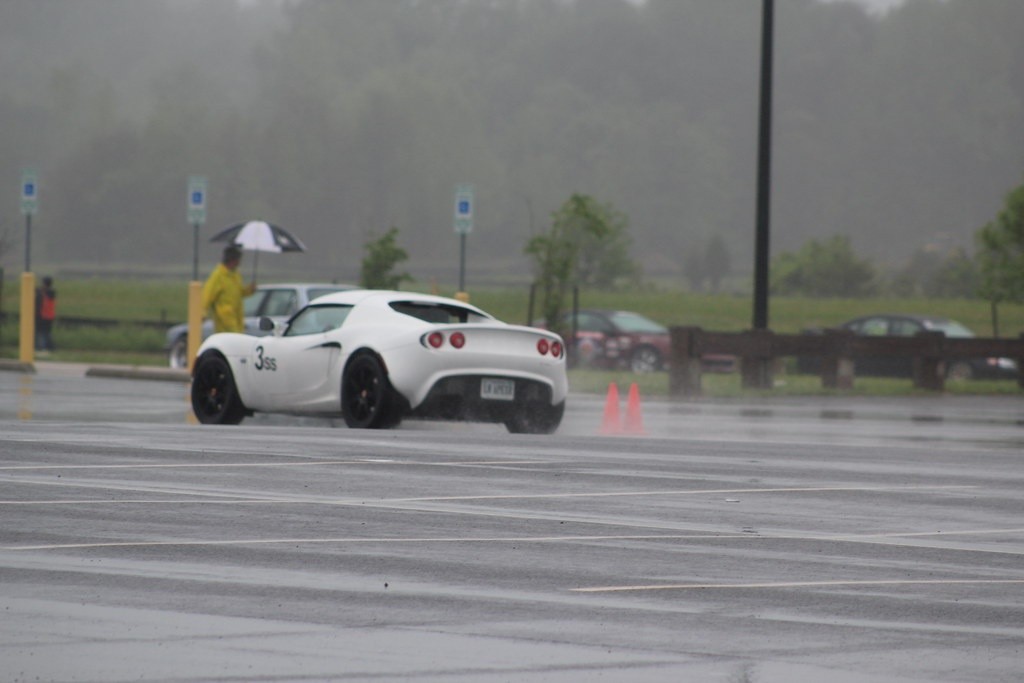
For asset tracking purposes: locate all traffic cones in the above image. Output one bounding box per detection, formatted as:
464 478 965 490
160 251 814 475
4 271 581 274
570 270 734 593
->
623 382 647 435
598 380 626 438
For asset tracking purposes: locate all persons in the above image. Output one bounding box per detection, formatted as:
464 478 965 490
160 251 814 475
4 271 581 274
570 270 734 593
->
34 275 55 350
200 246 258 336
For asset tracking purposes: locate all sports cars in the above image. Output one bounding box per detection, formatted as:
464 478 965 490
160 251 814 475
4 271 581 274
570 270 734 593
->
187 291 570 434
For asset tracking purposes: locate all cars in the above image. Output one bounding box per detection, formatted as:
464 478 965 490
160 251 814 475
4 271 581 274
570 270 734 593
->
797 313 1019 386
163 282 362 376
532 308 733 378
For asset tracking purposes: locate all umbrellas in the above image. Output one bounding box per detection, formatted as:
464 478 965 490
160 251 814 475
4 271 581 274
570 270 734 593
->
208 219 307 279
